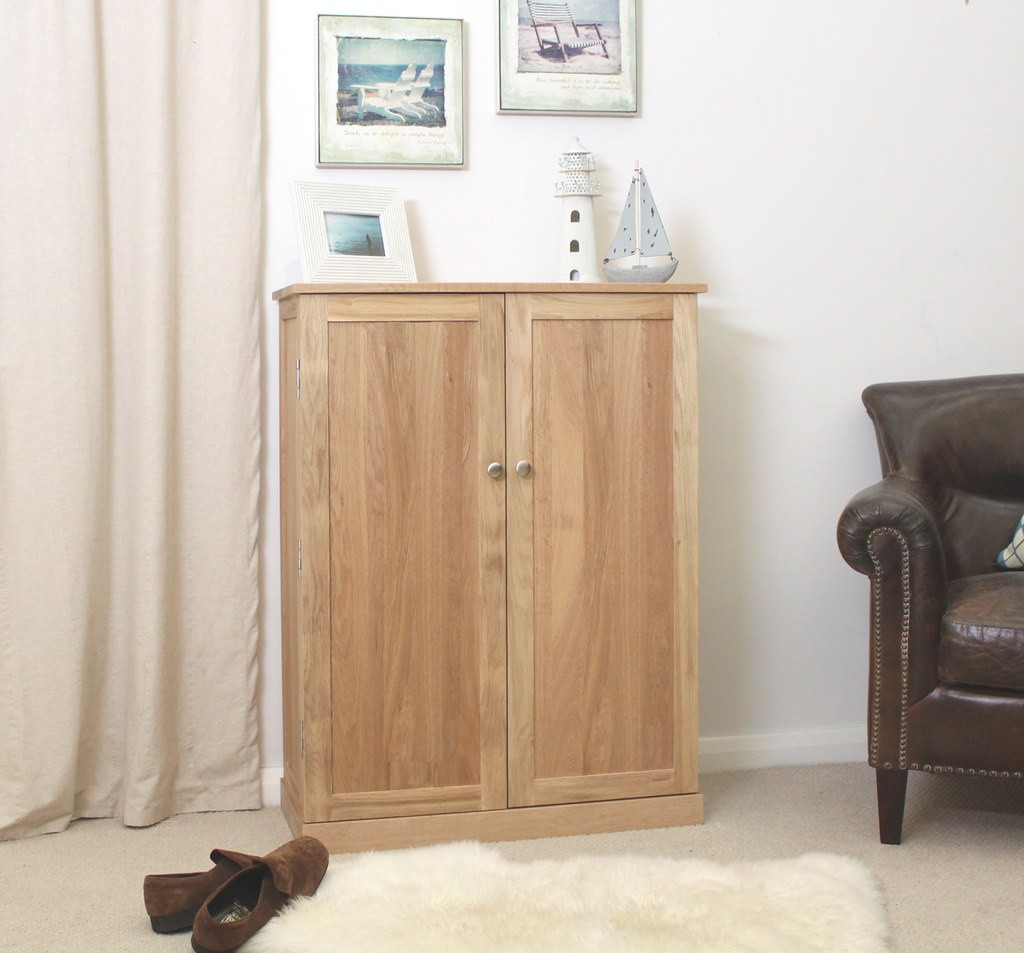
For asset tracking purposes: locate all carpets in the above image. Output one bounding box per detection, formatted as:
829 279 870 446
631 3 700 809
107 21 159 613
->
233 841 895 953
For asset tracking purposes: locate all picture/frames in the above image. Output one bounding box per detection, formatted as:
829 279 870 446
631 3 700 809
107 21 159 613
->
312 12 474 174
290 178 424 288
494 0 642 121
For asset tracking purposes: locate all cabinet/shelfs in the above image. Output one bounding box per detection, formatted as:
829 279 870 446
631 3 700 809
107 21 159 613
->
271 274 711 853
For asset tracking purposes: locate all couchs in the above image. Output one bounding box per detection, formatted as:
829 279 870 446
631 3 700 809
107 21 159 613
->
834 368 1024 850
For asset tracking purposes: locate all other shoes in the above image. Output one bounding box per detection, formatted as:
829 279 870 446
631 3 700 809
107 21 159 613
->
143 848 262 934
191 835 329 953
